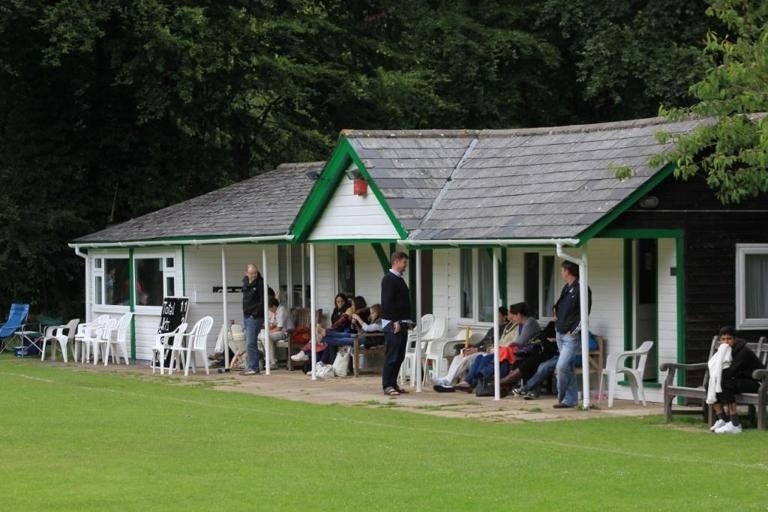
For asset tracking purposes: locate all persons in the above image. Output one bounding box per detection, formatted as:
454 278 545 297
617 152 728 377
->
316 304 385 346
473 305 558 396
478 302 542 386
105 264 116 303
512 329 599 399
257 296 296 368
241 263 276 374
380 252 417 396
291 293 347 373
452 304 518 391
307 297 366 375
119 279 144 304
551 261 592 409
706 326 764 433
432 306 509 392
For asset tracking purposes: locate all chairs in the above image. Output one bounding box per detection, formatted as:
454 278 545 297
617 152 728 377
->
396 313 604 398
169 314 214 375
600 340 652 408
268 307 385 377
0 303 133 366
152 323 188 375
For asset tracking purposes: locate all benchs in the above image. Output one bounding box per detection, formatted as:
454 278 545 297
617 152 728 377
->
660 335 767 430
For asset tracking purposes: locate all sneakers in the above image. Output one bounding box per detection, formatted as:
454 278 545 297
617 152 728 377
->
433 383 456 393
512 387 537 399
384 386 400 395
710 419 742 433
290 351 309 362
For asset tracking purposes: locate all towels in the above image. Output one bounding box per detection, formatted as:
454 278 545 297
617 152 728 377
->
705 343 734 404
